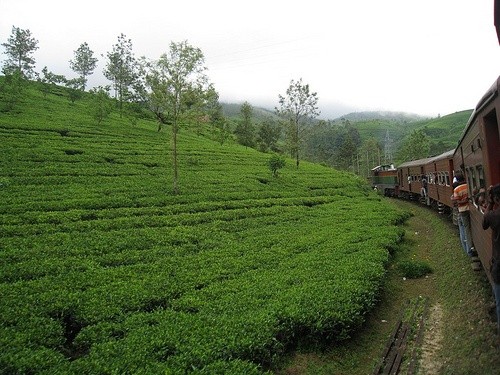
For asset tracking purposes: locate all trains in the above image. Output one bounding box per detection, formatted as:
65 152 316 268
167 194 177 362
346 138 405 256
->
368 76 500 330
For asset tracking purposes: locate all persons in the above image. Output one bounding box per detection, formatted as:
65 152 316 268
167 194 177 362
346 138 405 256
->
419 174 428 197
408 174 412 191
449 174 475 254
471 185 489 210
482 182 500 284
